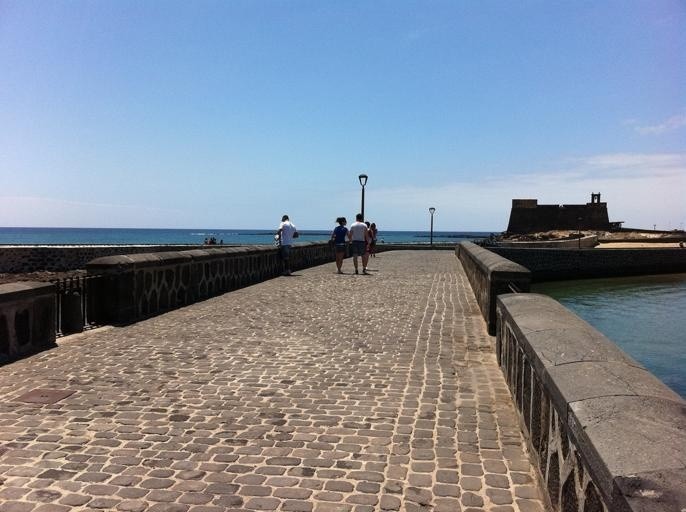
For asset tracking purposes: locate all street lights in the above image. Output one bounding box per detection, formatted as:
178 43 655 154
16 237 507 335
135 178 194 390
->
358 174 368 222
578 217 582 248
428 207 436 246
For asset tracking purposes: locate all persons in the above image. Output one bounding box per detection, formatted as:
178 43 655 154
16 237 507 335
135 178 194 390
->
679 240 683 247
274 215 296 275
365 222 377 257
204 237 224 245
348 213 369 275
332 218 351 274
